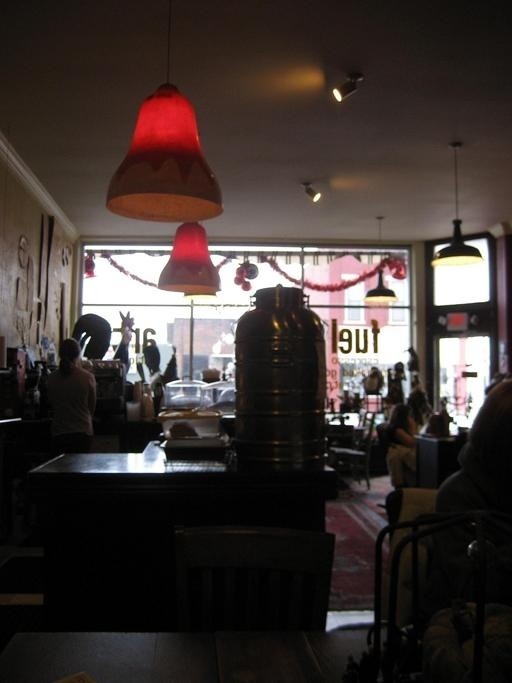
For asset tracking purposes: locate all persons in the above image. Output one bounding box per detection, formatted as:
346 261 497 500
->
42 336 97 456
381 403 420 491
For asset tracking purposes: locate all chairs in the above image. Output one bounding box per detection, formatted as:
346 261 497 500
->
386 487 444 630
375 422 389 445
173 523 336 632
330 408 373 491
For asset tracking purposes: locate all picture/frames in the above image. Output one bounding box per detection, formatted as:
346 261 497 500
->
16 277 27 309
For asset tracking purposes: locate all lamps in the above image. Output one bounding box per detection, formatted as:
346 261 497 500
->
157 222 221 292
332 70 366 103
431 140 483 269
363 215 399 300
301 180 321 203
105 0 225 223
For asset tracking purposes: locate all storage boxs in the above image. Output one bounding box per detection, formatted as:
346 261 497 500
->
159 407 223 444
165 374 215 407
214 378 237 413
